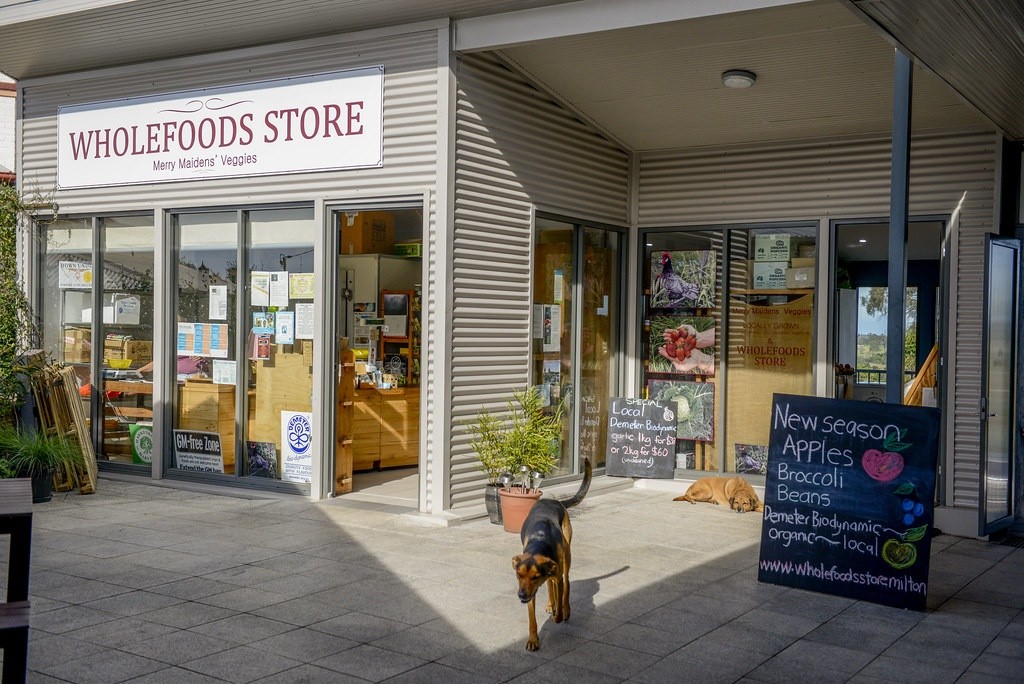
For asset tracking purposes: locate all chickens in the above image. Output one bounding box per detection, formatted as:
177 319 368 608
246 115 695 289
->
653 251 700 308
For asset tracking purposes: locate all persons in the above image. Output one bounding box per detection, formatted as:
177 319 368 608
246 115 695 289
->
135 316 208 382
658 325 715 375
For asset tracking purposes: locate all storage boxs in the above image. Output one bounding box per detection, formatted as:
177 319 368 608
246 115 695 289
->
82 293 140 324
393 243 422 257
675 452 694 469
791 256 815 267
198 297 232 326
16 349 45 370
383 373 398 389
64 329 153 368
340 212 395 253
787 268 816 288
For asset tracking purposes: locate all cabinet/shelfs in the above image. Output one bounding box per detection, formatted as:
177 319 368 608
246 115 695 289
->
378 289 421 386
352 389 419 469
58 261 198 402
180 377 235 473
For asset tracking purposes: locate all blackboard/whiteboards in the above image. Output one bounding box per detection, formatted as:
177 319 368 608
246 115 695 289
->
605 397 679 479
758 392 942 613
559 377 603 464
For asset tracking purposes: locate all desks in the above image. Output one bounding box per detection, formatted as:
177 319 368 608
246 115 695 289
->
0 478 34 684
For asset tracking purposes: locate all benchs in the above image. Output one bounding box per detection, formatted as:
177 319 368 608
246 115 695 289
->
0 600 30 648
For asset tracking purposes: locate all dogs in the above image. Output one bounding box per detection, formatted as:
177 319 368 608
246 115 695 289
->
673 477 765 514
512 457 593 651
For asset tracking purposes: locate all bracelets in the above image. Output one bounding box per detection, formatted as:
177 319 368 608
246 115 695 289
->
704 355 715 373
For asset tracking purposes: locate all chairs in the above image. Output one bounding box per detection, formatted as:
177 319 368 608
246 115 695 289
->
105 380 154 420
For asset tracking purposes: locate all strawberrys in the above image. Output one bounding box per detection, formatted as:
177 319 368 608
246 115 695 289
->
665 327 697 361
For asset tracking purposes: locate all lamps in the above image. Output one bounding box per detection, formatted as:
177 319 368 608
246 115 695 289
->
721 71 757 89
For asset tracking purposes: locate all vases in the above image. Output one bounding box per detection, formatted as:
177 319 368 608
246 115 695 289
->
838 375 854 400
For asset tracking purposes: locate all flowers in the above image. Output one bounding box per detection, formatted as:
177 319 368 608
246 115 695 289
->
836 362 856 375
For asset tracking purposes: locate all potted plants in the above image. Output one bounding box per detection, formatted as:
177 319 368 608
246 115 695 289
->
464 404 503 524
496 385 563 532
0 418 109 498
